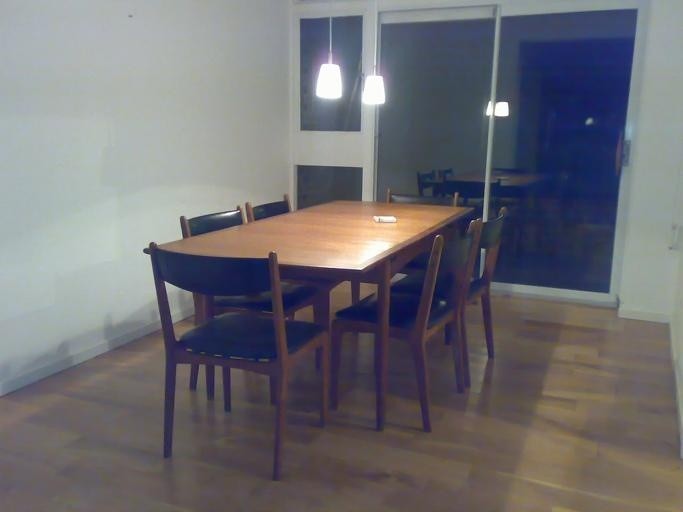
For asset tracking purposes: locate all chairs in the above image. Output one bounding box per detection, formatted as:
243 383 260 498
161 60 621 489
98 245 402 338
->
387 187 460 206
180 204 322 406
417 167 577 267
149 242 329 482
330 219 480 434
245 192 362 374
388 206 508 389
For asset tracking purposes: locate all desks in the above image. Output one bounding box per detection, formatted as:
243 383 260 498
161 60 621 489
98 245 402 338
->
145 200 476 428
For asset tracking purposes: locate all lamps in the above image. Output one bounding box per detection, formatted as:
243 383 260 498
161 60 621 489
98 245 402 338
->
361 0 386 106
315 17 343 100
